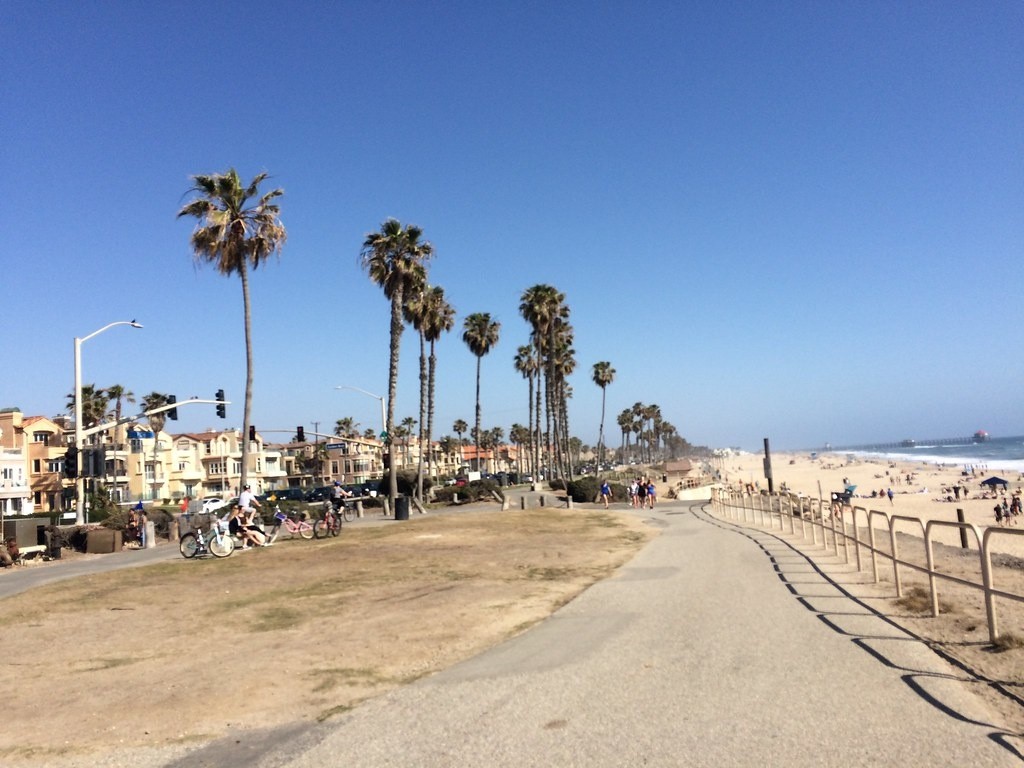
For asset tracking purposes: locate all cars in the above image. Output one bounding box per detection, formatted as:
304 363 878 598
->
482 472 544 483
306 487 332 502
267 488 305 503
573 465 604 474
198 498 225 514
445 478 456 485
342 487 363 497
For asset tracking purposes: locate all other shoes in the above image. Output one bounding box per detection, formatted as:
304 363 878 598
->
642 506 645 509
261 543 273 547
242 546 252 550
605 507 608 509
650 506 653 509
634 506 638 509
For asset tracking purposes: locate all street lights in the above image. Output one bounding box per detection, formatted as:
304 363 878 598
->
74 320 142 527
335 384 389 471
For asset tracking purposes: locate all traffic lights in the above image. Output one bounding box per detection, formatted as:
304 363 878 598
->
215 389 226 418
65 448 77 479
250 425 256 440
383 454 390 469
167 394 177 420
297 426 305 442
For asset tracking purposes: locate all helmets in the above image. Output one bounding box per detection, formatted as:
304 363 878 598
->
335 481 340 486
244 485 250 491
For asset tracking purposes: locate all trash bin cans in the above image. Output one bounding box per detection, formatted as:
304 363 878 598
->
395 497 409 520
663 476 667 482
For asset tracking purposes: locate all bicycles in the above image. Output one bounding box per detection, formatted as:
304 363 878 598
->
268 503 315 541
335 493 355 522
313 503 341 537
178 512 235 558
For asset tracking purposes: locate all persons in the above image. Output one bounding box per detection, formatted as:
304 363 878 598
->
330 481 348 514
229 485 272 549
887 488 893 502
994 495 1023 527
600 480 612 509
125 508 147 546
978 483 1008 495
631 475 656 509
1015 487 1022 493
952 484 969 500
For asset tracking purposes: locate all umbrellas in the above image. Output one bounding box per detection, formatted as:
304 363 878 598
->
982 476 1007 484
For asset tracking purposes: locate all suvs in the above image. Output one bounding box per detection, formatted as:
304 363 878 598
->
365 482 379 494
457 480 467 486
